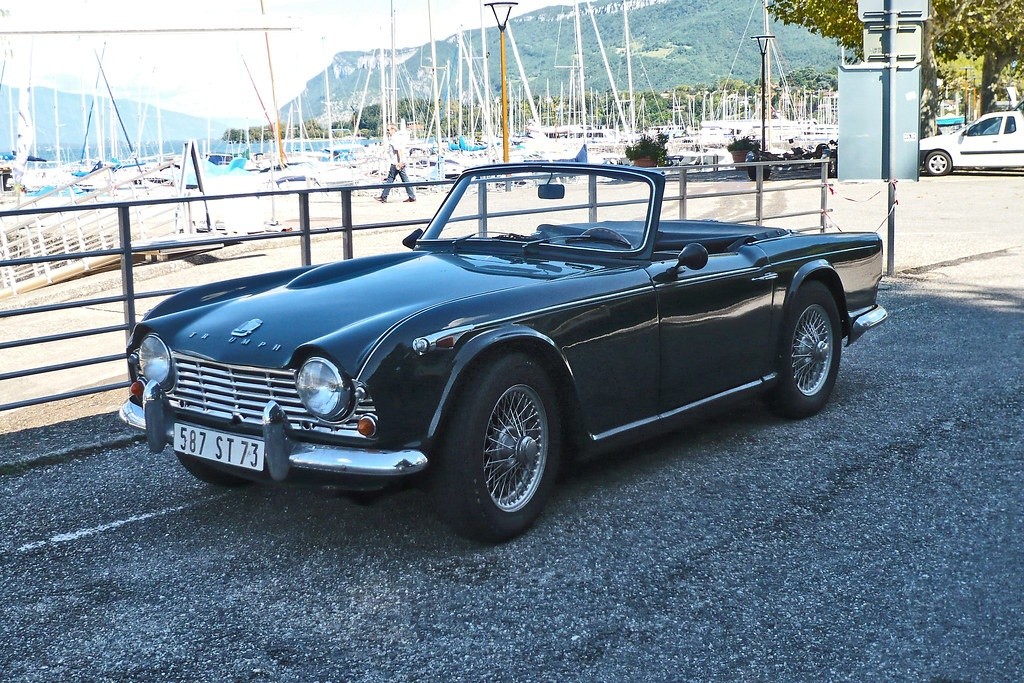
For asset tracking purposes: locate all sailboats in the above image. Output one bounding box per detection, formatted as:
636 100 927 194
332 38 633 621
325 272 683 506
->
0 0 837 162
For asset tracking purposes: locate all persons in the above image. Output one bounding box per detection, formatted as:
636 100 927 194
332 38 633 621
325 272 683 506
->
374 123 416 202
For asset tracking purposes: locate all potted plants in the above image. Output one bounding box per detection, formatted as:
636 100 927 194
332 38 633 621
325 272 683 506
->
625 132 669 167
727 134 760 171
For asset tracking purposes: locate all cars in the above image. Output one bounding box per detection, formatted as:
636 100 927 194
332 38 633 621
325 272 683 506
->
119 160 890 543
919 111 1024 176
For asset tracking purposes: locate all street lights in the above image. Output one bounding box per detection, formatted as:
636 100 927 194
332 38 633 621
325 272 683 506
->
484 2 519 161
750 35 774 152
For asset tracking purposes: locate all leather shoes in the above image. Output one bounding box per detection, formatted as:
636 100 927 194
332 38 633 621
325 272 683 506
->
374 197 387 204
403 197 416 202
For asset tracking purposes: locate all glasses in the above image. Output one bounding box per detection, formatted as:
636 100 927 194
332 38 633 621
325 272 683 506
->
387 129 389 131
